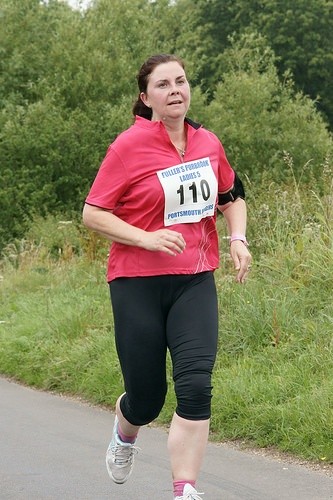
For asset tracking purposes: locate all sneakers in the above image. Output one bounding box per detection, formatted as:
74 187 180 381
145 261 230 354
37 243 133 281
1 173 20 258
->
173 483 204 500
106 414 137 484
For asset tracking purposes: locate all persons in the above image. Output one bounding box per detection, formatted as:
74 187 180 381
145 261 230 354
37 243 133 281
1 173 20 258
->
83 53 253 500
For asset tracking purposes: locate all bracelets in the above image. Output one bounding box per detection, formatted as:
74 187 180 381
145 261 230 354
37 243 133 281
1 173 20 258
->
230 235 248 246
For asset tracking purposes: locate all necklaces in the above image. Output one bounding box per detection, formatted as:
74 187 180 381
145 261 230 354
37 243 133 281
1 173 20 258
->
175 146 185 156
